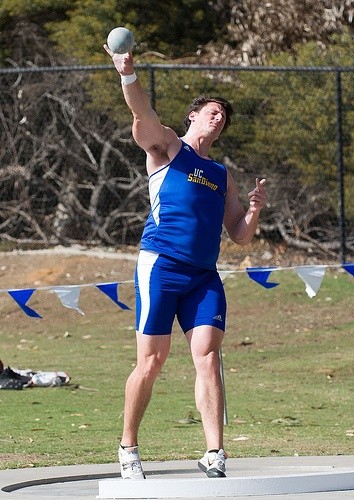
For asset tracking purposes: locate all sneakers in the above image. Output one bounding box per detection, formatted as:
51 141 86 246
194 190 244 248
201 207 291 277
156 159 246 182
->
198 448 228 478
118 444 146 480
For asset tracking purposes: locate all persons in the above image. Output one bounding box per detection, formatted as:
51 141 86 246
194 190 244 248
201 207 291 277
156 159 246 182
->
102 44 267 479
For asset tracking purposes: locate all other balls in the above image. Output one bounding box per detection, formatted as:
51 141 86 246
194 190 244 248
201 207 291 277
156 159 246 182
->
107 26 135 54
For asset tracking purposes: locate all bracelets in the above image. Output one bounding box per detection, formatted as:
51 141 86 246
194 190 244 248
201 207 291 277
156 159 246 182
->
121 73 137 85
